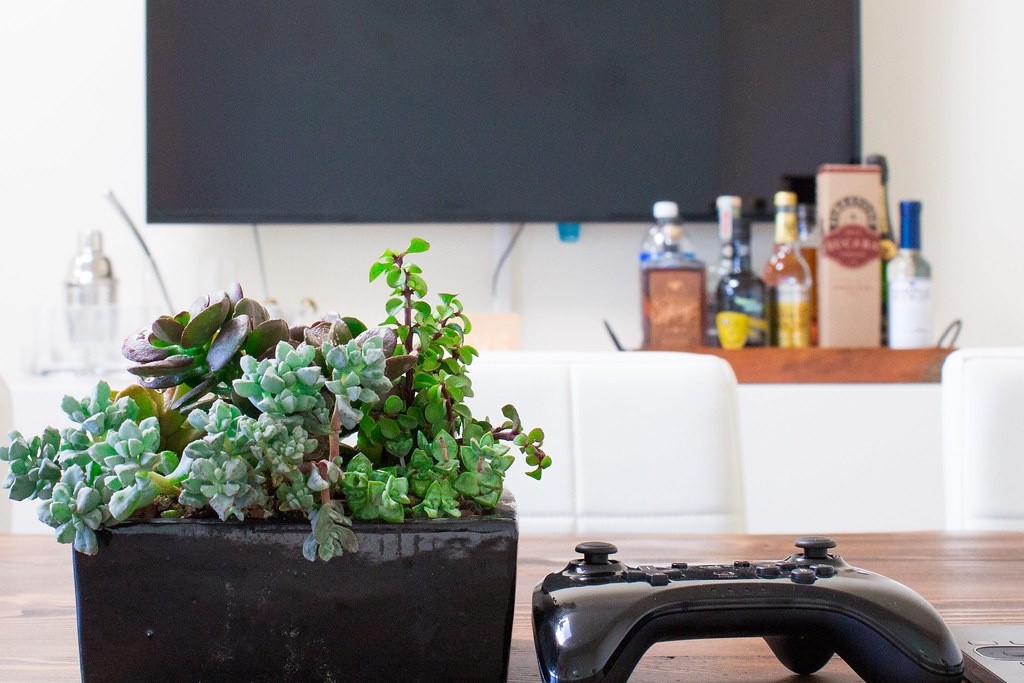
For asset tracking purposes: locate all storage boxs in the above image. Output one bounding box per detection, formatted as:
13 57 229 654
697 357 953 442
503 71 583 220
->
814 164 883 349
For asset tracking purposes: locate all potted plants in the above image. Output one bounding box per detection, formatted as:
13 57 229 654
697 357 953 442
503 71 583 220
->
0 237 551 683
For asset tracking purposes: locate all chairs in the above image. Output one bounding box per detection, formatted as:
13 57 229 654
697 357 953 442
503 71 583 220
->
940 348 1024 531
443 349 742 535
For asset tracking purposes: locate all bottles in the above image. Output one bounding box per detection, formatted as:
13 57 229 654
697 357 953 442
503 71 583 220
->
640 156 934 349
61 225 116 374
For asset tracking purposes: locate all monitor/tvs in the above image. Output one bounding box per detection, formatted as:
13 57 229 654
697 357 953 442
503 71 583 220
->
147 0 863 226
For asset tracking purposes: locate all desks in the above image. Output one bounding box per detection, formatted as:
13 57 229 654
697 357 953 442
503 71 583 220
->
0 534 1024 683
